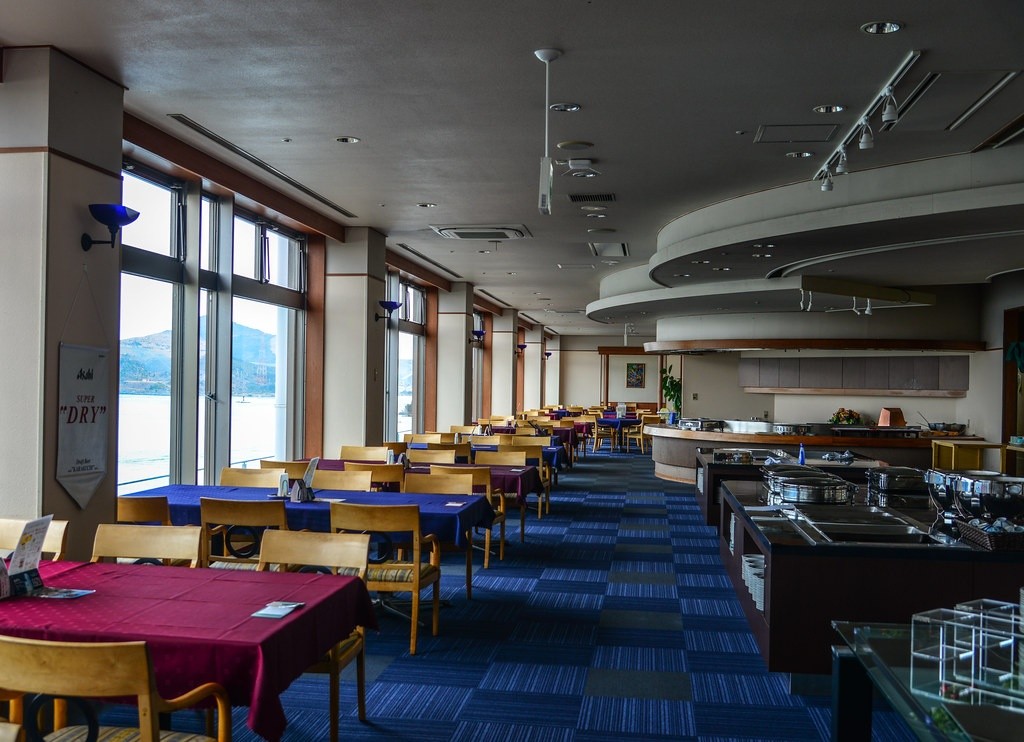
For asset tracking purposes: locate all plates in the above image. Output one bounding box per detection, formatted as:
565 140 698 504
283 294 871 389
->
717 485 764 611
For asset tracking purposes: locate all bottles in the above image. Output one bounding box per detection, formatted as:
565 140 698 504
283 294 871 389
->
798 444 806 465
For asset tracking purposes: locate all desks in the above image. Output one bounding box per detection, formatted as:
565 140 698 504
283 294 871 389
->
596 419 623 453
0 558 381 742
932 439 1006 473
574 422 593 456
287 458 544 543
617 418 642 446
392 443 569 514
121 484 497 635
460 426 579 472
830 620 1024 742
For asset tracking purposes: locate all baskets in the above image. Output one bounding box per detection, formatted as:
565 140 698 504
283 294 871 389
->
952 516 1024 551
728 513 734 558
741 553 764 611
698 467 705 496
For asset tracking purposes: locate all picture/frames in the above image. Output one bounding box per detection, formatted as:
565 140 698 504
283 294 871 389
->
626 363 645 389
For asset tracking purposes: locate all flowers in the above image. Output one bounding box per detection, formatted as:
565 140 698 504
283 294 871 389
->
828 408 864 426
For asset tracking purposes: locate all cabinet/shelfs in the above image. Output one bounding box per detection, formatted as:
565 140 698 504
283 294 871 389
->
738 356 969 397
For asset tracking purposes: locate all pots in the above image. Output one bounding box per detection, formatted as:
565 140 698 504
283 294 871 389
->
916 411 966 432
865 466 926 493
759 463 858 505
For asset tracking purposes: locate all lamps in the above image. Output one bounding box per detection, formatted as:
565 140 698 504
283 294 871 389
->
542 353 551 360
852 297 872 316
514 344 526 355
799 289 813 312
375 300 402 322
469 331 486 344
81 204 140 252
817 78 898 192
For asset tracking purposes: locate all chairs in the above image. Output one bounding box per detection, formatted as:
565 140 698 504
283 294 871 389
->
0 404 661 741
